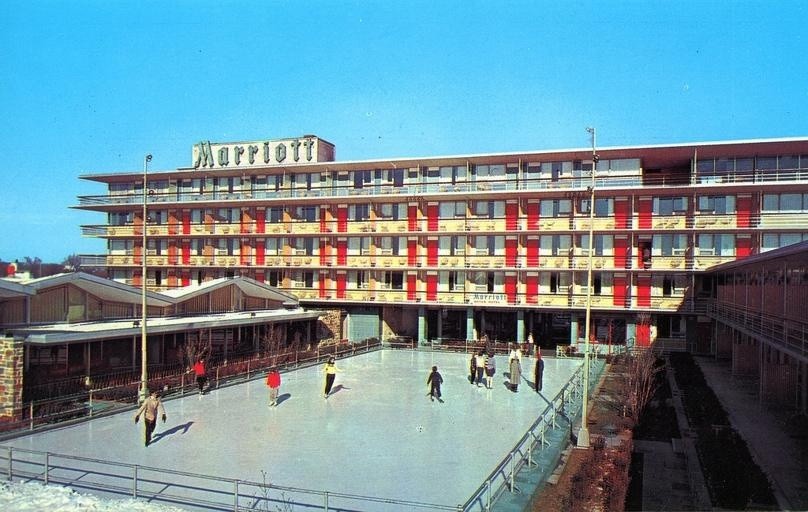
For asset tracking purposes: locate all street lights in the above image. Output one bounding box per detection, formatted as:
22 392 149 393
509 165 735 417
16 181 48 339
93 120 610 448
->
575 124 599 449
135 154 153 421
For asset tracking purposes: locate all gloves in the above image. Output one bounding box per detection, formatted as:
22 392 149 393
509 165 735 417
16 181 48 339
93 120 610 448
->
135 416 139 423
163 414 166 422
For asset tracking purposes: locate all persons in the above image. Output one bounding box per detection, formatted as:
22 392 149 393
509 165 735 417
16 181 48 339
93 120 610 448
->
483 351 496 390
469 352 479 384
134 387 167 447
164 340 211 369
532 353 544 392
506 345 524 372
508 358 521 394
527 331 534 357
426 366 444 402
322 357 344 399
642 245 652 262
624 245 631 269
266 368 280 407
478 333 489 344
475 351 485 387
185 356 207 396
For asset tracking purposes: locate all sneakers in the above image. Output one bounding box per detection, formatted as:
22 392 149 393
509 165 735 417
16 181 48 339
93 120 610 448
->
269 402 277 406
324 394 329 398
471 382 492 389
431 396 442 400
